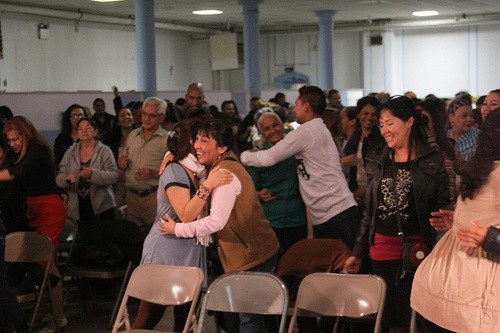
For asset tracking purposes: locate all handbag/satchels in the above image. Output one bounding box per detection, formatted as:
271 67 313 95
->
397 232 438 280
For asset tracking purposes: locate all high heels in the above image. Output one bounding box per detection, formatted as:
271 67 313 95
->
41 316 67 333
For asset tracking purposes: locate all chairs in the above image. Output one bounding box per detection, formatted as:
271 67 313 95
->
60 220 145 329
274 239 358 333
112 264 205 333
288 272 387 333
194 271 289 333
4 231 53 329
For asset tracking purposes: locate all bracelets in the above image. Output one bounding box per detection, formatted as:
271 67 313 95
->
195 183 212 201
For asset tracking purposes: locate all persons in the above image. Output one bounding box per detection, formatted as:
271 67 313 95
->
0 82 500 332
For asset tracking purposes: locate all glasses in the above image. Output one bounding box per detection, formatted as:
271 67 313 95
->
141 111 163 119
69 114 85 120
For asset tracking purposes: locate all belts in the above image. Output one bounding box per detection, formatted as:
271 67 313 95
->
129 185 158 197
